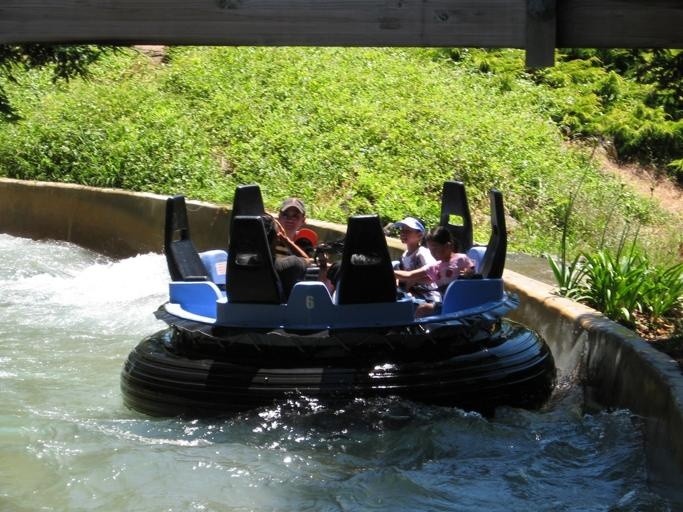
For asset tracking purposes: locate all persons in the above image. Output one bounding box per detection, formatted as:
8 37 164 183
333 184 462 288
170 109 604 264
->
262 213 311 304
291 228 332 281
415 268 482 317
310 239 345 305
393 225 476 298
396 217 440 303
275 197 318 260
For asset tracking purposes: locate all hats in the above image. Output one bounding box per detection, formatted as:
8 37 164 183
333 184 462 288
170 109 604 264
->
292 228 318 246
279 198 307 216
395 217 426 236
254 212 278 240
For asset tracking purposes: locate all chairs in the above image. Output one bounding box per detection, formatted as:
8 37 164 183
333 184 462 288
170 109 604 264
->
440 180 473 253
477 188 508 276
230 184 264 217
332 214 400 304
225 214 287 304
164 194 213 281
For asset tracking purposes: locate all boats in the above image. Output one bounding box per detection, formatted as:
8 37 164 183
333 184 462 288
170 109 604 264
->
121 181 559 422
